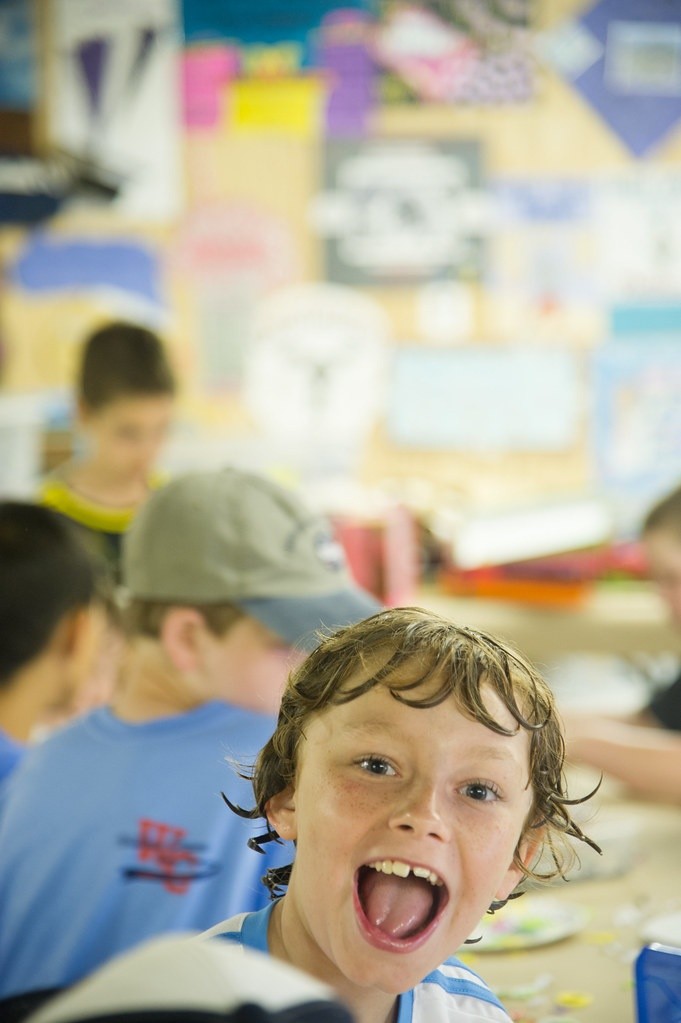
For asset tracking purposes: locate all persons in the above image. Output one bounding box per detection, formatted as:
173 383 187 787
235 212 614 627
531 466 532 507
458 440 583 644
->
0 494 108 744
557 483 681 807
176 605 603 1023
1 467 386 1002
29 320 180 579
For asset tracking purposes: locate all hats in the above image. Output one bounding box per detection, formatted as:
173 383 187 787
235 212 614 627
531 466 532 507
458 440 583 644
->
126 464 381 650
